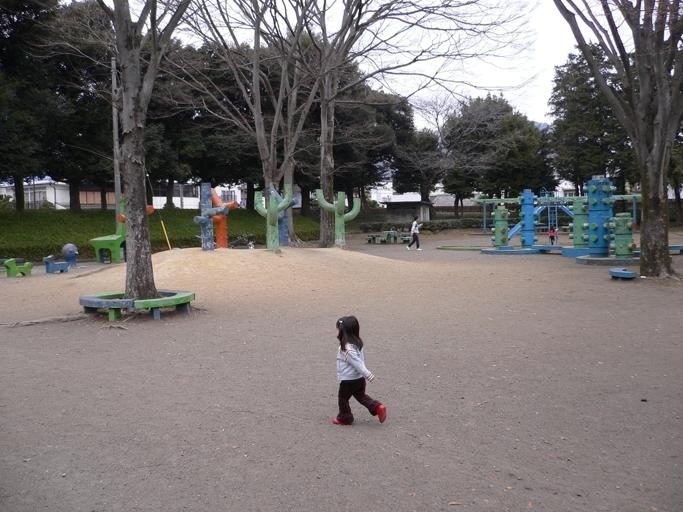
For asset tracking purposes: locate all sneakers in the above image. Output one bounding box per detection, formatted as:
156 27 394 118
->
406 245 411 251
332 417 343 424
376 405 386 423
416 248 423 251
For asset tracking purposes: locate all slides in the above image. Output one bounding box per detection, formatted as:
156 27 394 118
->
508 205 546 241
558 204 574 218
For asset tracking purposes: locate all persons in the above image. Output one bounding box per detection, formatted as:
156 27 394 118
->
331 315 386 426
547 226 555 246
405 215 424 250
